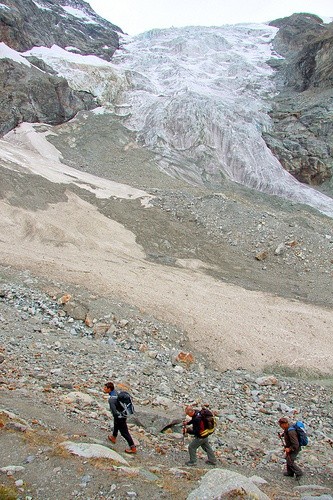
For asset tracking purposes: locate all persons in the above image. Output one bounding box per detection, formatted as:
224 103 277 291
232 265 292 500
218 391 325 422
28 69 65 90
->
277 418 303 481
103 382 137 453
181 405 218 466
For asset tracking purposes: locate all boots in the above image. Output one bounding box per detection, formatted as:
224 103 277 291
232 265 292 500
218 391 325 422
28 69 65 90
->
125 447 136 453
108 435 116 443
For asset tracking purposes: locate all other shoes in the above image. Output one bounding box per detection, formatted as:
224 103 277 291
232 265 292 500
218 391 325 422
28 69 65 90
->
283 472 294 477
205 461 216 465
295 473 303 481
185 462 196 466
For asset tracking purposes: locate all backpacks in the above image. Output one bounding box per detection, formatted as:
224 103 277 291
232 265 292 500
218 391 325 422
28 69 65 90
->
193 407 214 435
288 421 308 446
109 390 134 415
